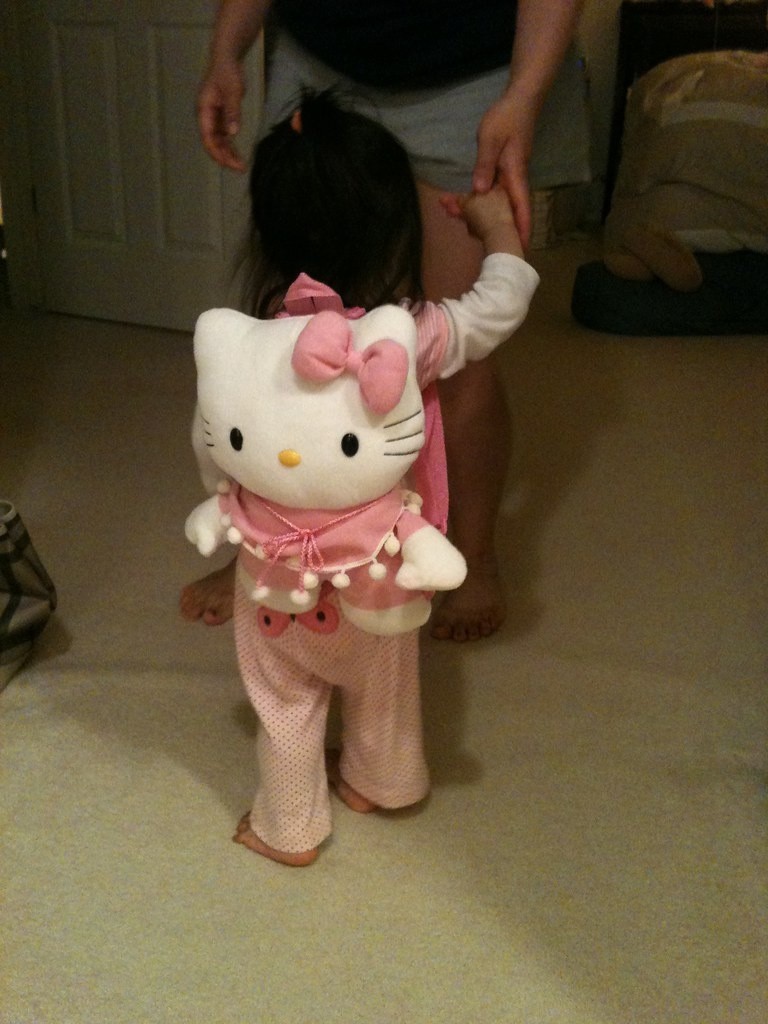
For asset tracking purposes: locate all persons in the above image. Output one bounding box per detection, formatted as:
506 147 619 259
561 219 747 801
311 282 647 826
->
193 88 540 866
179 0 585 641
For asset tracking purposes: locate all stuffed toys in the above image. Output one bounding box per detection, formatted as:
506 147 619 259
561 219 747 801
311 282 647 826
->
184 307 466 635
